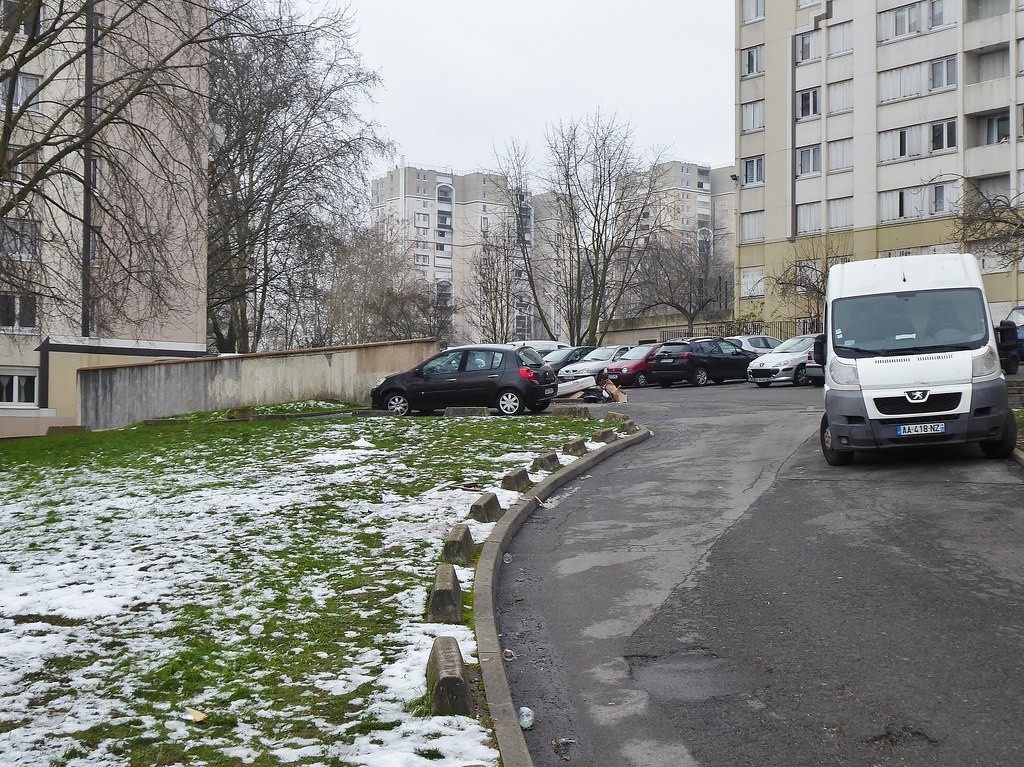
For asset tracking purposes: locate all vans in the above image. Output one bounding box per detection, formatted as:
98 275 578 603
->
506 340 571 357
819 253 1017 465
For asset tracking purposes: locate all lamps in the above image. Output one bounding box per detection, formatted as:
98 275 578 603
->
730 175 739 180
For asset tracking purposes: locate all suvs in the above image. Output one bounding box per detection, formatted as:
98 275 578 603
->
724 335 782 355
997 306 1024 363
652 336 759 387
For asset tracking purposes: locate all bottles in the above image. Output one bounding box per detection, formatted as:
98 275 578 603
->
518 707 534 728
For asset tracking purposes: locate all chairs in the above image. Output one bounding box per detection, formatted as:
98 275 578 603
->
466 351 494 371
845 302 962 343
752 339 760 348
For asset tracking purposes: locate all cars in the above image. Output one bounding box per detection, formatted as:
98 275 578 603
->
370 344 558 416
557 345 635 383
747 332 824 387
535 345 597 381
602 343 662 387
